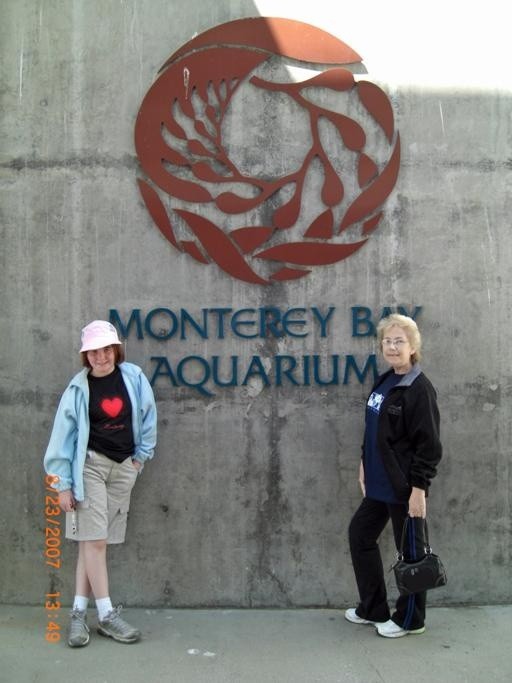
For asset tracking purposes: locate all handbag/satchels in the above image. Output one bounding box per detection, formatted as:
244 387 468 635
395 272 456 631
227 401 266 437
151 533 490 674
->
393 552 448 597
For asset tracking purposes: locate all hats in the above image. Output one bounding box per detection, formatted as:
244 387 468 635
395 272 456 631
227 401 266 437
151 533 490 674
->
79 321 123 355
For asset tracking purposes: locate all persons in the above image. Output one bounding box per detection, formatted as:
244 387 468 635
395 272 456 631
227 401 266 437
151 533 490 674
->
43 319 158 647
342 310 443 639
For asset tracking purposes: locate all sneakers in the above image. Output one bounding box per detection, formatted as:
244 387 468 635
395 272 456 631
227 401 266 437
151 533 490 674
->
96 604 140 643
378 618 424 637
346 608 381 624
69 605 91 646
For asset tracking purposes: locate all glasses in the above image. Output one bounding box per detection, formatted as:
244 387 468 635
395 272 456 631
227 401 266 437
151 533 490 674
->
383 338 406 346
